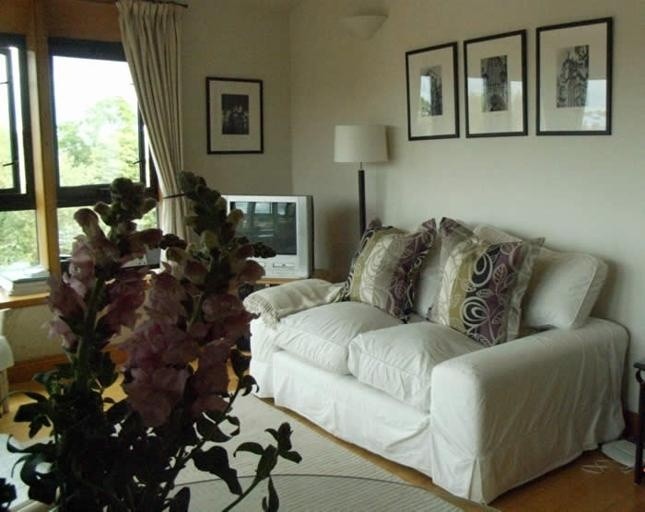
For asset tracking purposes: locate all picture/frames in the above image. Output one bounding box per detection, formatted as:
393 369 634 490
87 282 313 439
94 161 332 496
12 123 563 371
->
206 78 264 155
404 42 458 141
536 15 613 137
462 30 528 136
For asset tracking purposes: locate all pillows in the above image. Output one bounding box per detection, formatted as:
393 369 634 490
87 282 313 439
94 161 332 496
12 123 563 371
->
426 218 547 345
343 219 445 323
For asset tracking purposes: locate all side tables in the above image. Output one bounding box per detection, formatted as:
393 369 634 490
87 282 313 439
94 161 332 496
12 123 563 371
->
230 275 300 356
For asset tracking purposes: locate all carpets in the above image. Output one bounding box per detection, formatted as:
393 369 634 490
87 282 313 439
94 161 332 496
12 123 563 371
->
23 388 466 512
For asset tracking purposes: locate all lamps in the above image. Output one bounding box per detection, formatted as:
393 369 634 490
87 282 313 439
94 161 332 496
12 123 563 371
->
333 125 390 243
338 16 384 40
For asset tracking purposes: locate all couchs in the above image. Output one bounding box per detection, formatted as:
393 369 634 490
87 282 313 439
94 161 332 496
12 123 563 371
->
235 226 630 505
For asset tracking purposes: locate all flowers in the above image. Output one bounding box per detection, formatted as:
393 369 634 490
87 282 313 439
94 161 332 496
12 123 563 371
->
1 173 302 512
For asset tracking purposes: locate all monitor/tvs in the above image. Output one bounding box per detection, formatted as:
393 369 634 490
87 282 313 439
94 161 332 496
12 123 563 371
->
222 195 313 280
